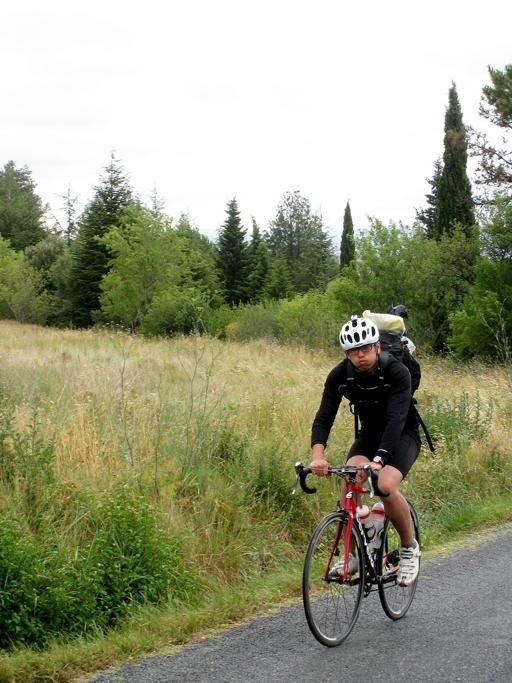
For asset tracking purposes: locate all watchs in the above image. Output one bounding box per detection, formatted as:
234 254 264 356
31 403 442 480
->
373 456 384 467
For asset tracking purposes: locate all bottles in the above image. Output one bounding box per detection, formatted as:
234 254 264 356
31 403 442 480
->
370 502 385 549
356 500 375 537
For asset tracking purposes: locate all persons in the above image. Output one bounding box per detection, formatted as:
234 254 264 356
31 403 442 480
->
308 317 423 587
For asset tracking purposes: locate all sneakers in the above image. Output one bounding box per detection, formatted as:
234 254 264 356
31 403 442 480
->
327 553 358 576
396 538 420 587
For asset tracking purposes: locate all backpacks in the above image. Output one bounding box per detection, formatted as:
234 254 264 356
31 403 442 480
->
344 332 421 400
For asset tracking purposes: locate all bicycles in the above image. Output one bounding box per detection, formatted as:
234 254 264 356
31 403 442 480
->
292 459 424 646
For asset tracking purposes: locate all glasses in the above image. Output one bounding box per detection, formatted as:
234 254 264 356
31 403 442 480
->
345 342 377 356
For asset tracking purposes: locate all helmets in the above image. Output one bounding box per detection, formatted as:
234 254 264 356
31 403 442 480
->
338 317 381 352
